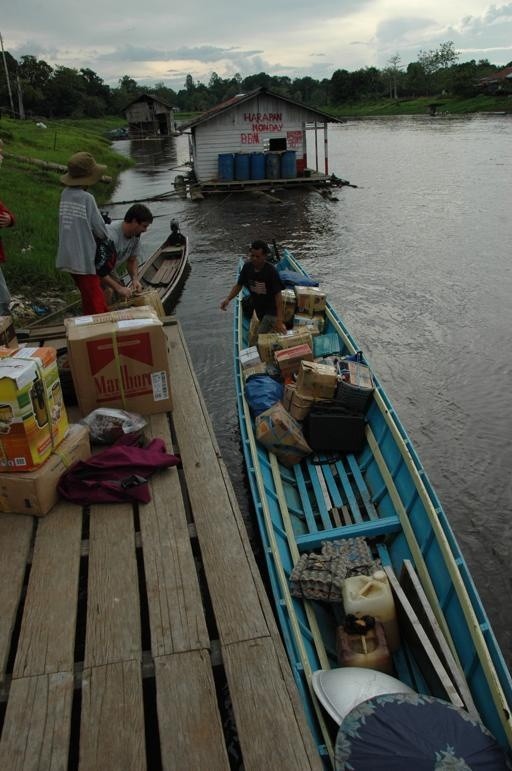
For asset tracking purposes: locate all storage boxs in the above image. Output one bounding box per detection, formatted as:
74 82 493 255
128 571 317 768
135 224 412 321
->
0 315 19 349
0 423 93 516
64 306 173 417
238 286 373 469
114 290 167 323
0 347 70 472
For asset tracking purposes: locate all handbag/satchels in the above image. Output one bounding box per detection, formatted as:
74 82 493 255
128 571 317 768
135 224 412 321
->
95 238 117 278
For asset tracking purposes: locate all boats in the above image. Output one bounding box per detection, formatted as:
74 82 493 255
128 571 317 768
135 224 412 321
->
233 237 512 771
15 217 189 344
175 85 349 201
117 92 176 140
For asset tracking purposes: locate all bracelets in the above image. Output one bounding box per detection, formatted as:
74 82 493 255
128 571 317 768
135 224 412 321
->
277 320 282 322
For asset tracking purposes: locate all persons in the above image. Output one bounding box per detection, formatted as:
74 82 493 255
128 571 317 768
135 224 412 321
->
220 239 287 335
101 204 153 306
54 151 114 313
0 201 17 316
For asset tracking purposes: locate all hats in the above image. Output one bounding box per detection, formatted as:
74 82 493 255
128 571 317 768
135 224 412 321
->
60 151 108 187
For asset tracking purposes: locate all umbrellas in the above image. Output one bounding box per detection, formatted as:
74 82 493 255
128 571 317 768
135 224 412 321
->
335 692 509 771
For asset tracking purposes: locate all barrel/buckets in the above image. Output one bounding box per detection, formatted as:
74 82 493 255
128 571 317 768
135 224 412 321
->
336 619 394 674
218 150 296 183
341 571 399 652
